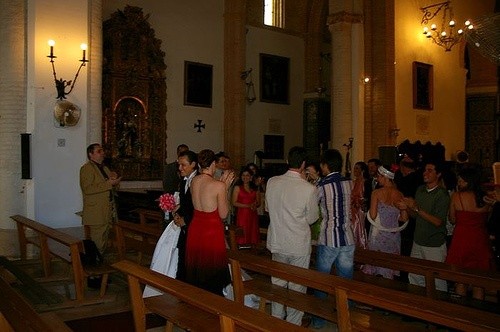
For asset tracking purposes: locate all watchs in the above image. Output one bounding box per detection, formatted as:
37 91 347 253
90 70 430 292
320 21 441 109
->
415 208 421 215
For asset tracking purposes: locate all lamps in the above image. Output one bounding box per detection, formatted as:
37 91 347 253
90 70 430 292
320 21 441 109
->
46 39 89 101
241 68 257 104
420 1 474 53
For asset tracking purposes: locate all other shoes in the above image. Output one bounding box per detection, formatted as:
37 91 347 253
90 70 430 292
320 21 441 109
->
302 319 311 328
88 276 112 289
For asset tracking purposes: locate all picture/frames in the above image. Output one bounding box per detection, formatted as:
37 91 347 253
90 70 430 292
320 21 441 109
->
184 60 214 109
412 62 434 111
258 53 291 105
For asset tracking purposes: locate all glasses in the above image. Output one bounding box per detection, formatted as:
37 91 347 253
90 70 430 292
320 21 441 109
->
95 149 105 153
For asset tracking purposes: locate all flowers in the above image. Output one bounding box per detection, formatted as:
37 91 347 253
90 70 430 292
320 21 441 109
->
157 193 185 227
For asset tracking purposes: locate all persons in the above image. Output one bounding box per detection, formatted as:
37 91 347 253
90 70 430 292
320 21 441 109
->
162 145 267 298
80 143 121 257
303 149 500 330
265 146 320 326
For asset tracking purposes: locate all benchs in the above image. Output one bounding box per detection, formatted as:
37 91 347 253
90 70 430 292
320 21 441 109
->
0 210 500 332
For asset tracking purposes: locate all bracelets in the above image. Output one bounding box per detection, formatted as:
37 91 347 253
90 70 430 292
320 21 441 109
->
315 177 319 181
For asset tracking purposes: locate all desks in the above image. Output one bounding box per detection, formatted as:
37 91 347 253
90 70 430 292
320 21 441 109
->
116 186 164 222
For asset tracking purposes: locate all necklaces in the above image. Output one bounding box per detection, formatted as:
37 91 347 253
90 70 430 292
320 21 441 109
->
200 172 213 177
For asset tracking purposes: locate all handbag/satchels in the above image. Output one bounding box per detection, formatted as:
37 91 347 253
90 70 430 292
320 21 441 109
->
68 240 103 265
